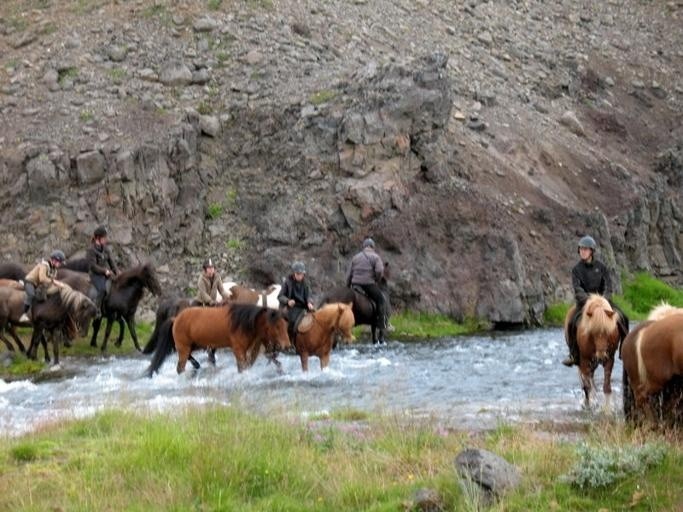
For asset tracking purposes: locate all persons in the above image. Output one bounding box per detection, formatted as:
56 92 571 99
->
191 257 229 307
277 260 315 353
561 235 628 367
18 249 67 324
85 226 120 321
345 238 385 330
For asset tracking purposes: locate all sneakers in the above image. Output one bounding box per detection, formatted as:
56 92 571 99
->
563 355 577 365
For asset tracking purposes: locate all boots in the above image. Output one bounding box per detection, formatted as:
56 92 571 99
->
20 304 30 321
95 291 105 318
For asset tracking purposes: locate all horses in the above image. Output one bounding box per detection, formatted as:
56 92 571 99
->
56 261 161 353
143 282 356 380
564 292 621 410
0 256 102 365
316 261 391 350
621 300 683 434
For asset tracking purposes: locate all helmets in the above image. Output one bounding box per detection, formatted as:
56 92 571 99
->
363 239 374 248
50 250 66 266
577 236 597 252
94 229 107 236
292 262 305 273
203 260 214 267
